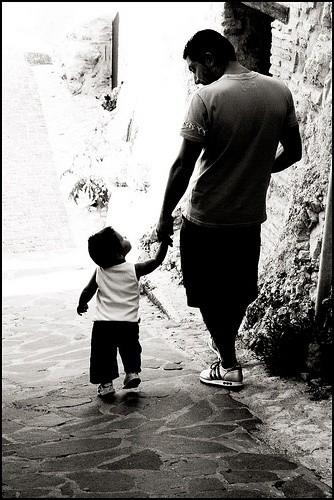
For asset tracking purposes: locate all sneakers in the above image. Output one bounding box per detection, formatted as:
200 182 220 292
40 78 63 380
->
207 338 224 362
200 358 244 386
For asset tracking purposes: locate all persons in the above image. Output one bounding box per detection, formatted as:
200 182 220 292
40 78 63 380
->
76 226 170 399
155 29 302 389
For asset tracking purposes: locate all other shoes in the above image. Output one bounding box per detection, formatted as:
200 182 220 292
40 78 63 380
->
122 372 141 389
97 382 115 395
